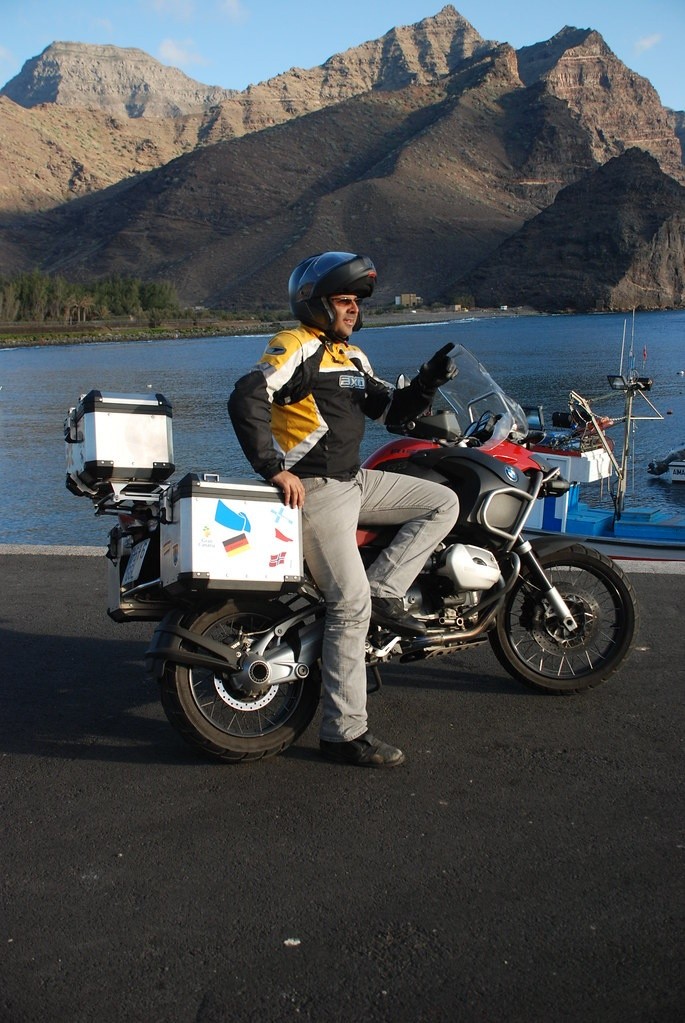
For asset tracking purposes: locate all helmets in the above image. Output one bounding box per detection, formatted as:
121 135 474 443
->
288 251 378 330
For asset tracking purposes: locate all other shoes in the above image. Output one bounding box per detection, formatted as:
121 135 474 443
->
320 731 404 766
369 595 428 638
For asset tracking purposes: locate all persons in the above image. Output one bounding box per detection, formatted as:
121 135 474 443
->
227 251 460 767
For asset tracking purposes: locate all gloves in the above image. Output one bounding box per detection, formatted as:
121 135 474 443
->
417 341 459 392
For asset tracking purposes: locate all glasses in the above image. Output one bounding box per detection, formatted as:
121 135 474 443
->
330 297 363 308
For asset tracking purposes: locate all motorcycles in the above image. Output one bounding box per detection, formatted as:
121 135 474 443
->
102 341 641 765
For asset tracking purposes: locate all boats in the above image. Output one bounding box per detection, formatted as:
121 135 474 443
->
523 302 685 543
647 443 685 482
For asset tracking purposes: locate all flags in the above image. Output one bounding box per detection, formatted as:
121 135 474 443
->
642 344 648 360
